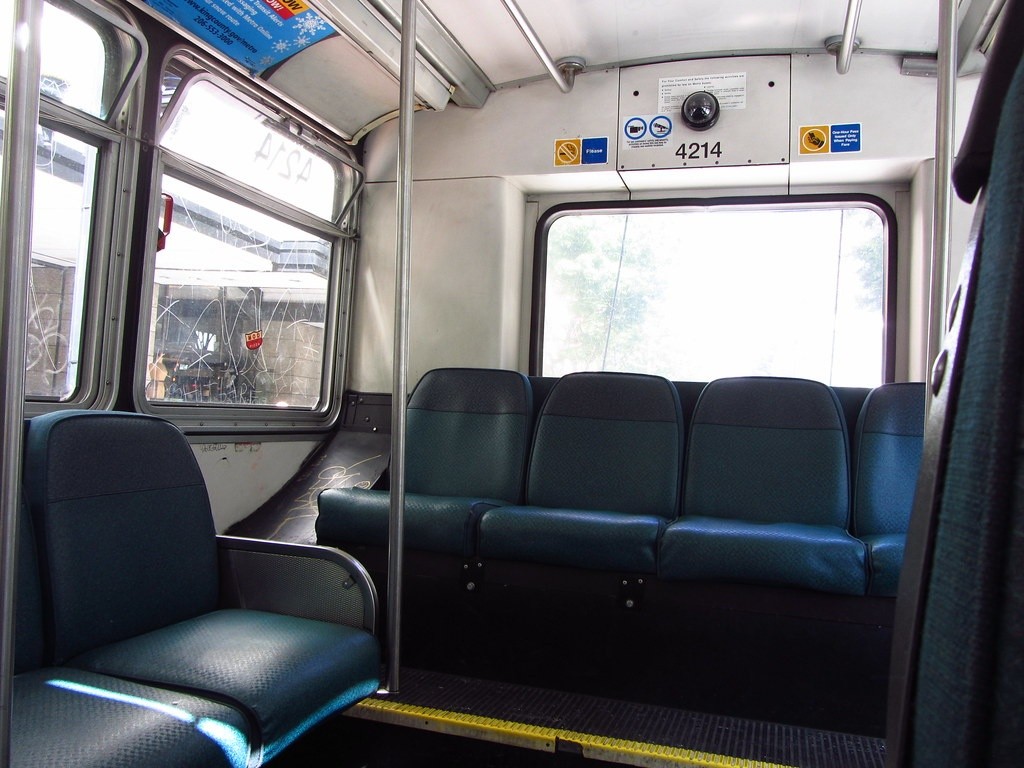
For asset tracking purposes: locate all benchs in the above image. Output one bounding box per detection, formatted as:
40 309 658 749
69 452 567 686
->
316 367 927 737
3 407 383 768
